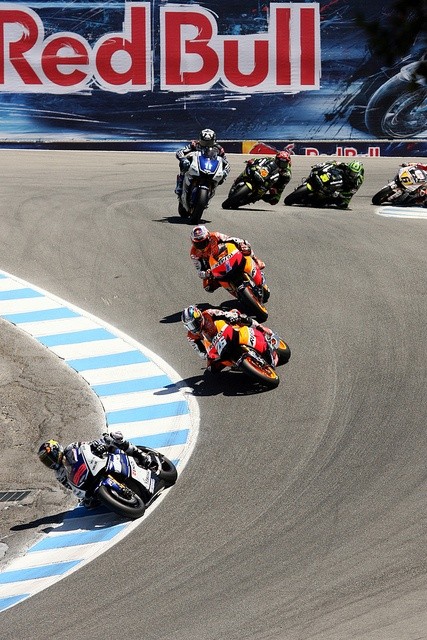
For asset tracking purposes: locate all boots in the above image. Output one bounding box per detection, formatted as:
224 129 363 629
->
252 255 265 270
175 175 184 194
248 318 272 337
125 441 152 469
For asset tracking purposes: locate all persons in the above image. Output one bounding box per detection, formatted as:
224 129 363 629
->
235 150 292 205
180 304 273 382
174 128 231 196
189 225 266 294
310 160 364 209
407 162 427 205
37 430 152 511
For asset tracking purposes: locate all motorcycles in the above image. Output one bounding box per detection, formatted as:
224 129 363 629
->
220 159 279 210
178 148 229 222
364 57 426 139
371 163 426 207
202 308 290 387
283 165 345 205
206 243 271 321
65 431 177 517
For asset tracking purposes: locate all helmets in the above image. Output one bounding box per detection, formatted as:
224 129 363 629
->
181 306 204 336
190 225 210 249
275 151 291 168
347 160 363 180
38 440 65 470
199 129 217 147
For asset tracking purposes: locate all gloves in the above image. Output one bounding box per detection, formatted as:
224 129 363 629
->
205 269 211 277
96 444 107 455
180 157 190 169
228 316 239 325
